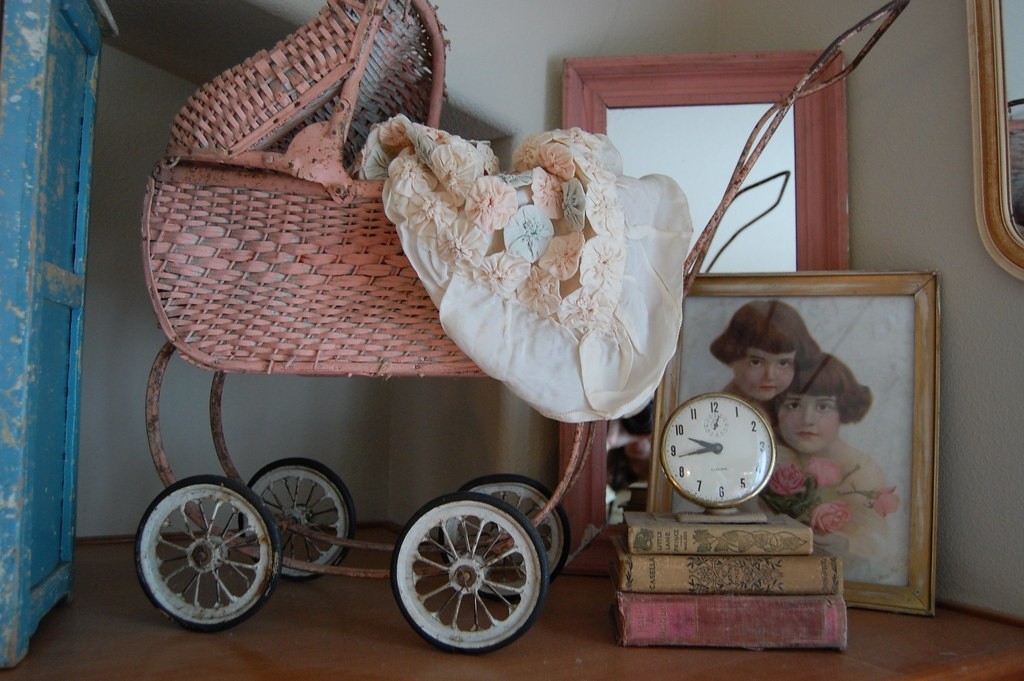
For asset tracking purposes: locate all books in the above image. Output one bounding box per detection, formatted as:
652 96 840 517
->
623 510 814 555
618 553 846 593
621 598 848 646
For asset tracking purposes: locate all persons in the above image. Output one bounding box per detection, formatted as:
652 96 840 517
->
710 300 890 586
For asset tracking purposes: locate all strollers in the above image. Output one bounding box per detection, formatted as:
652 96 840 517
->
133 1 694 657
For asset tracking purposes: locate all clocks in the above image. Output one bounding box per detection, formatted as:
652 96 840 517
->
660 393 777 524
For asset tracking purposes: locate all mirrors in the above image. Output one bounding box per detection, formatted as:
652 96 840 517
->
563 50 852 572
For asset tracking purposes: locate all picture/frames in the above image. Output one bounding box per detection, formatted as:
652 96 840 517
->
647 269 940 617
